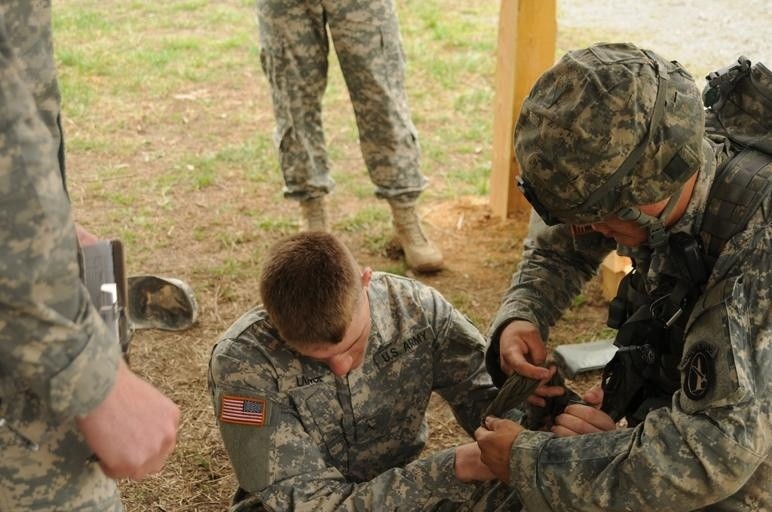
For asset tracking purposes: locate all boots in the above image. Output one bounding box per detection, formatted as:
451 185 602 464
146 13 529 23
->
299 196 331 234
386 196 443 273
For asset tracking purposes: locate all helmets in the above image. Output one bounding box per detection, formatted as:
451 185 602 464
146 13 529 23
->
514 41 706 228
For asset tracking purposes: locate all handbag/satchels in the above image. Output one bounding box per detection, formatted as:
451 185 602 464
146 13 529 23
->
695 57 772 289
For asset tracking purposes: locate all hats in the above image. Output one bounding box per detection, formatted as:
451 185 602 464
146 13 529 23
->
125 275 199 332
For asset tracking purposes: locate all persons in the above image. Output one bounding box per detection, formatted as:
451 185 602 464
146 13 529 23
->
0 0 180 512
258 0 445 273
473 42 772 512
206 231 616 512
